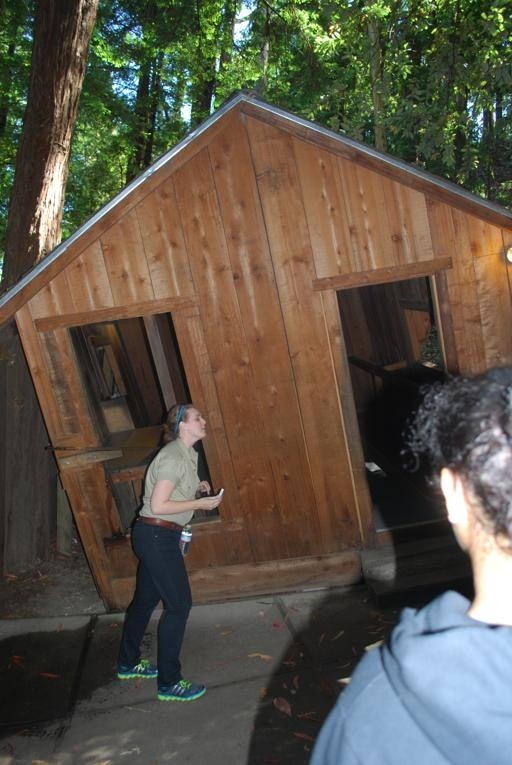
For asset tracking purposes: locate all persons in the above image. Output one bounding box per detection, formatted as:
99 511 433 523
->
307 364 512 765
116 403 223 702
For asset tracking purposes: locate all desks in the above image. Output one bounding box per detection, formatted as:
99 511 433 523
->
104 423 208 518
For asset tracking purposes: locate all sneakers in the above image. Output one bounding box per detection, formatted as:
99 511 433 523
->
118 659 159 678
156 680 206 701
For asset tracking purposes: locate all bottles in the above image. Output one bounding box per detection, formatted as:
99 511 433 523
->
178 524 192 555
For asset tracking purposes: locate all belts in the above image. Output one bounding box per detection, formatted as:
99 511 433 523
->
138 511 184 531
